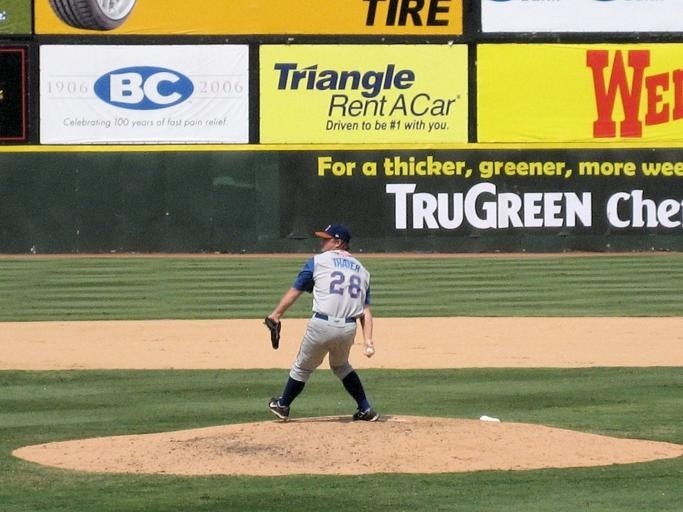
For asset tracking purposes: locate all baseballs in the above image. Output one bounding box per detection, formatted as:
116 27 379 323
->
367 347 374 354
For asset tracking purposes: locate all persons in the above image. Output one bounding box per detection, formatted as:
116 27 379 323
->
261 224 378 421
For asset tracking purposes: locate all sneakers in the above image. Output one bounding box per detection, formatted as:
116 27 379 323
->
268 398 290 419
353 406 379 421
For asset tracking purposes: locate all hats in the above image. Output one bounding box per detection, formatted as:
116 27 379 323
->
315 223 351 242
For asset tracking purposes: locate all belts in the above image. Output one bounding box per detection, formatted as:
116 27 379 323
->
315 313 356 323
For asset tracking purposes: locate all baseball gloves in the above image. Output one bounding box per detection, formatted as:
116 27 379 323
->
265 317 281 348
